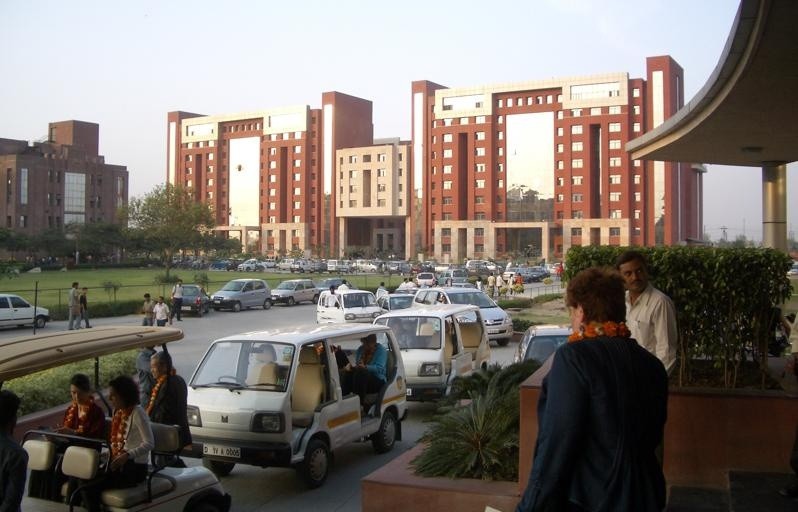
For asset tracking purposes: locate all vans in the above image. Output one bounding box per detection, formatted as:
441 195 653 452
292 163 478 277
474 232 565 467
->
372 304 490 403
178 322 408 489
411 287 514 347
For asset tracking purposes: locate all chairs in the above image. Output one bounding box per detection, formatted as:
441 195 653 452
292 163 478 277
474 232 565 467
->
243 344 278 390
287 346 326 426
459 322 481 360
428 321 452 373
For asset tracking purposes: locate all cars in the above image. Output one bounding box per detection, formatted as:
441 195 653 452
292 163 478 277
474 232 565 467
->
0 293 50 329
511 323 574 367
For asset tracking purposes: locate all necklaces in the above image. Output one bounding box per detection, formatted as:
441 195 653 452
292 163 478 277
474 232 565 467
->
145 375 168 415
64 404 91 432
568 321 631 342
110 408 133 458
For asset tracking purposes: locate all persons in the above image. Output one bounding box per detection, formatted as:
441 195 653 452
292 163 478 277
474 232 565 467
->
513 264 670 511
152 295 171 326
0 389 29 511
343 334 389 411
29 374 107 500
495 273 505 296
169 279 184 324
619 250 680 376
405 277 417 290
66 281 82 330
66 376 156 512
324 285 340 308
486 272 497 299
514 273 524 285
377 282 386 289
140 292 155 326
75 286 93 328
475 276 485 292
337 279 349 289
507 274 515 294
146 351 194 468
398 278 408 290
135 344 159 408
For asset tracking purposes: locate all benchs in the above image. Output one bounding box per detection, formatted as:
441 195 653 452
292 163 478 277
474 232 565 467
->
61 415 181 508
20 433 100 512
342 349 395 382
